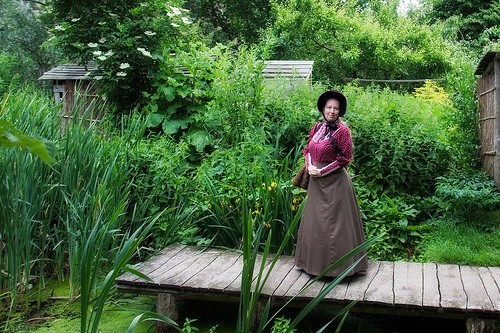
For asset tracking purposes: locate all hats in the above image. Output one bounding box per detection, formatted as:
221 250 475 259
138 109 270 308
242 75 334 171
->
317 90 347 124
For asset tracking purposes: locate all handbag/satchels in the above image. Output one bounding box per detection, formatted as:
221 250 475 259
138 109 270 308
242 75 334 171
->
291 164 309 189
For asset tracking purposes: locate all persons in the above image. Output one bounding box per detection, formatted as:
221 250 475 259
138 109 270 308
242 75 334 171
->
291 90 369 277
